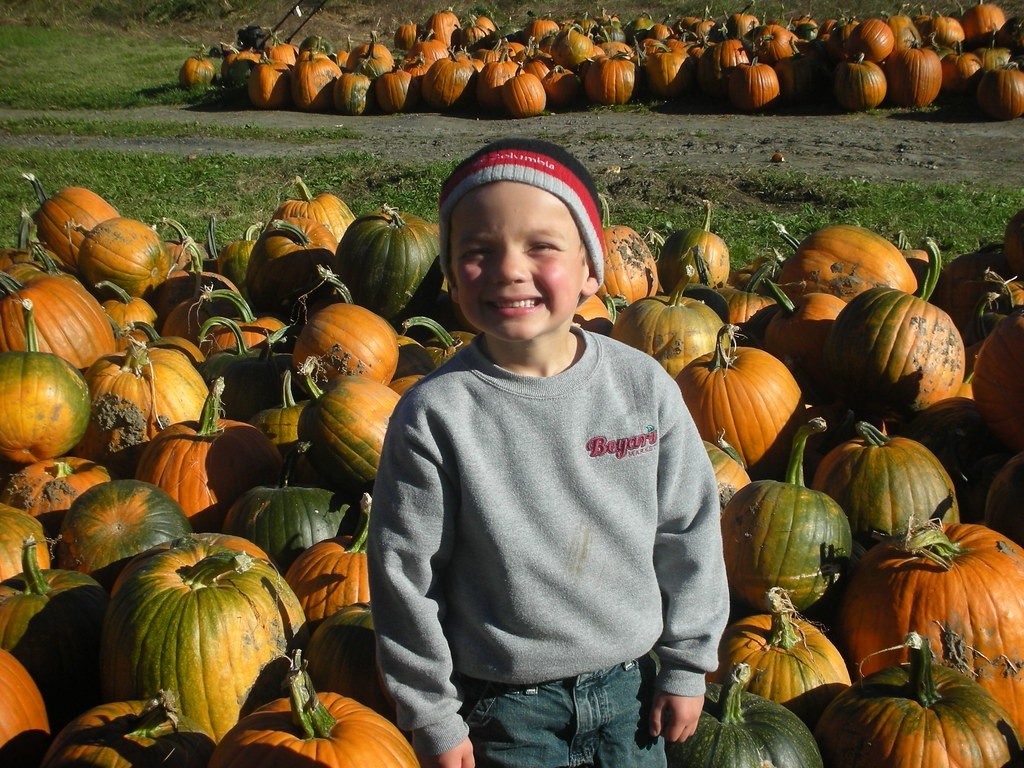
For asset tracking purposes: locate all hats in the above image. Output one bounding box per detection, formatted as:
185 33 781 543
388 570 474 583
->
437 140 607 309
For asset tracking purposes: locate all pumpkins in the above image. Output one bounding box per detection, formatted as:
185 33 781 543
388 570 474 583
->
573 195 1024 768
181 0 1024 129
0 170 483 768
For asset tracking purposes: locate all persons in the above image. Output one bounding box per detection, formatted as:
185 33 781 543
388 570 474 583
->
367 137 731 768
